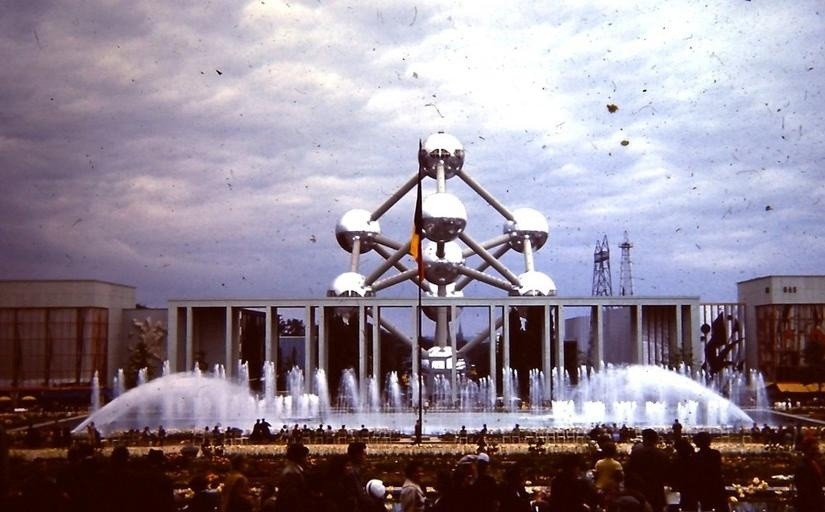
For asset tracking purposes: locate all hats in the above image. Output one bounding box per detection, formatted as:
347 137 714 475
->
365 479 387 502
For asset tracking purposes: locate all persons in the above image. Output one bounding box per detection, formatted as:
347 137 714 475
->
2 370 825 512
